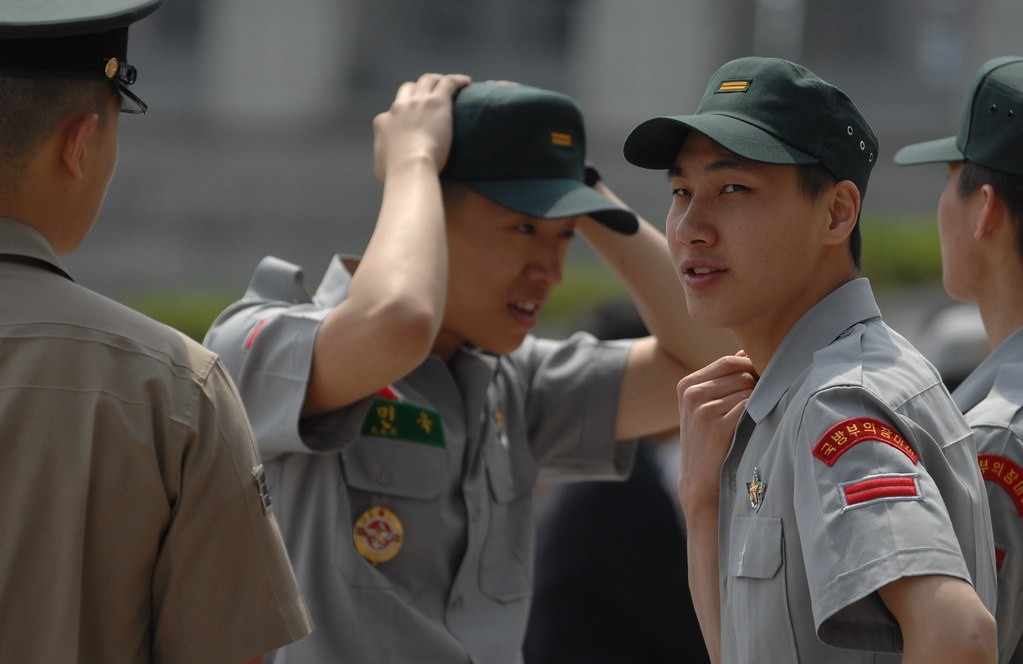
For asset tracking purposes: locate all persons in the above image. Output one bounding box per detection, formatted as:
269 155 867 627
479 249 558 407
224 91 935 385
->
623 55 999 664
203 71 744 664
1 0 313 664
891 54 1023 664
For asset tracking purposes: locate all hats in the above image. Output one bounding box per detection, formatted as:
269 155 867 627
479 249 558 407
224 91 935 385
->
892 55 1023 175
0 0 162 114
436 81 639 236
622 56 880 204
